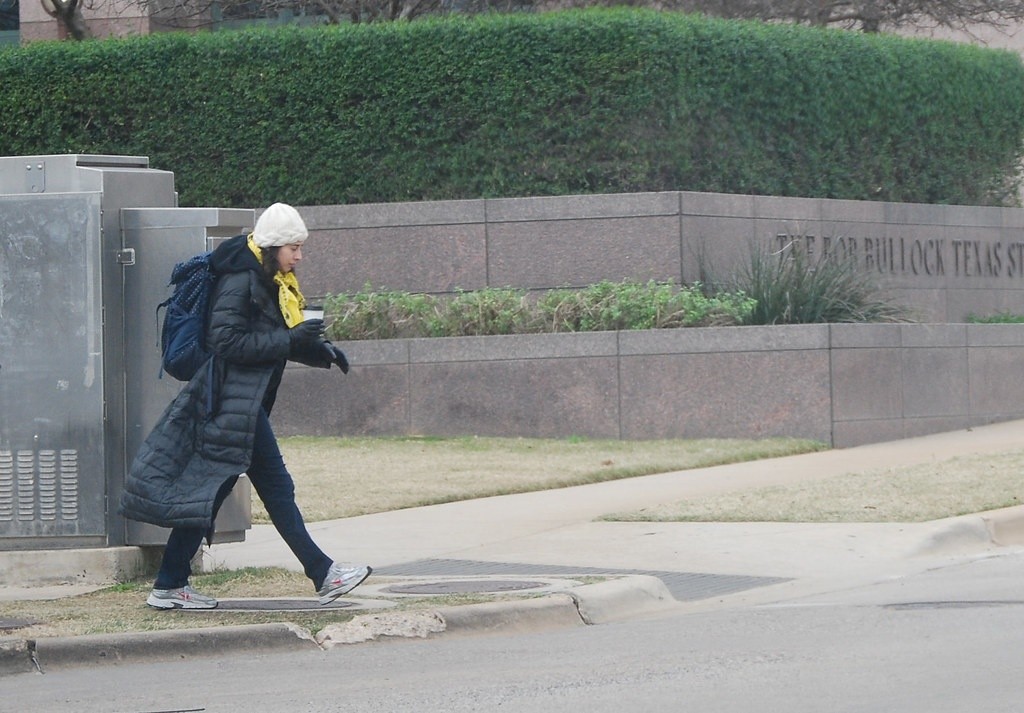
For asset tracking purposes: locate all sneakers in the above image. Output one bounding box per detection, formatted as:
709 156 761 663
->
315 564 372 606
147 586 217 611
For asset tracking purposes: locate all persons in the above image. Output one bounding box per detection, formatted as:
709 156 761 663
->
117 203 373 610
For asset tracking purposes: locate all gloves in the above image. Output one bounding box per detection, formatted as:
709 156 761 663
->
290 319 325 351
292 341 350 375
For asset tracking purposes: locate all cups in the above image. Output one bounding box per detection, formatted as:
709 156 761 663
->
300 305 325 322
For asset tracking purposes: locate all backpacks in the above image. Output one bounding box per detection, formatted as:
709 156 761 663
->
150 253 213 380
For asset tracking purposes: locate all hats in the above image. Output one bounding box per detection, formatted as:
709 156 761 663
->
254 202 307 250
